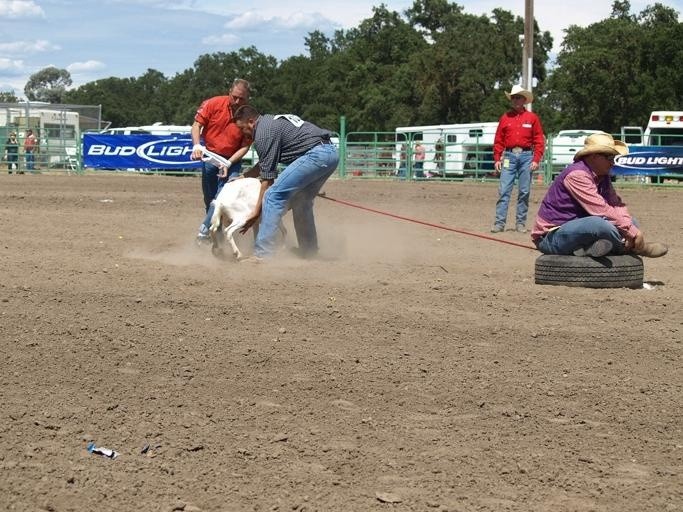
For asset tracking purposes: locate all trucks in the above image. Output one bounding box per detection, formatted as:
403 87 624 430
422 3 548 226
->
44 120 199 176
618 109 683 185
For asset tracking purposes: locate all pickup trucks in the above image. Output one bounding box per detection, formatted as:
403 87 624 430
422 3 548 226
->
544 125 617 185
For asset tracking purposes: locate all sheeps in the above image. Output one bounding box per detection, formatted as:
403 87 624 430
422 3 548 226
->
208 176 287 261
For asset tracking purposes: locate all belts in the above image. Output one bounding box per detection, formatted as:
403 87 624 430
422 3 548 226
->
313 137 335 148
504 146 533 154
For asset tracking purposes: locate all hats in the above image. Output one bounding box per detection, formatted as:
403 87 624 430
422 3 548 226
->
573 132 629 163
504 85 534 105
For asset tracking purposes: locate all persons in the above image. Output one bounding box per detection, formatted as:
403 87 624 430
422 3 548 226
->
21 129 37 171
352 139 446 181
191 79 252 242
227 105 340 265
530 132 669 259
4 132 24 175
490 85 545 233
462 145 547 186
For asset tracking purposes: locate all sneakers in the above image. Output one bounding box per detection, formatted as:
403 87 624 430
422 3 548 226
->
627 238 668 258
489 222 529 235
193 235 211 250
570 240 612 258
287 244 320 260
239 255 272 268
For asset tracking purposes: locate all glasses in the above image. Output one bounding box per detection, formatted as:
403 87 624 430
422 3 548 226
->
596 152 616 162
510 95 525 100
